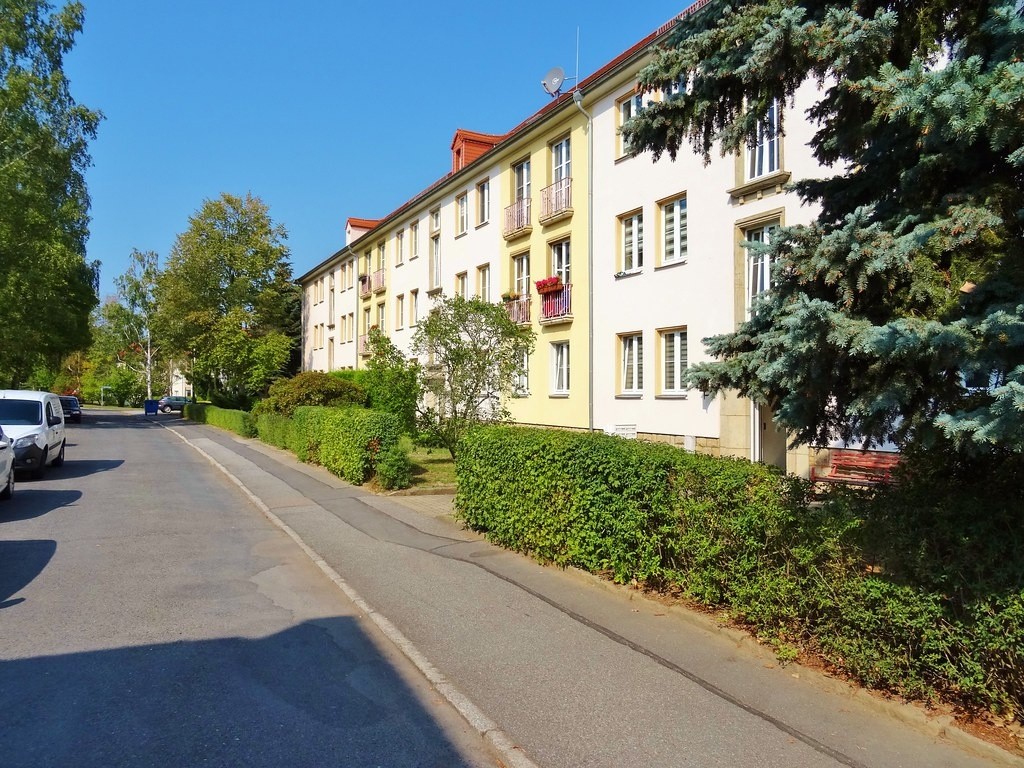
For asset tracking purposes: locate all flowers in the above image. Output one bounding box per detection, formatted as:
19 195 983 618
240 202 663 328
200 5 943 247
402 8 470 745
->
501 291 521 299
532 274 561 289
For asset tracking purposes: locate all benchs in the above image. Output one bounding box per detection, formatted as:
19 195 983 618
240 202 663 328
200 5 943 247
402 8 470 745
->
810 453 901 499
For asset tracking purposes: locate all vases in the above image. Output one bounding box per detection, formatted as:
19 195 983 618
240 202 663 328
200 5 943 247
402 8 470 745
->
539 284 563 295
503 297 519 302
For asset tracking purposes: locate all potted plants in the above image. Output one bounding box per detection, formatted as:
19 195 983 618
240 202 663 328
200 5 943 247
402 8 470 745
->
358 274 366 282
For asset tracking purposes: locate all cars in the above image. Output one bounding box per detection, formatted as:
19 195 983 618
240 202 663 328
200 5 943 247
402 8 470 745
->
58 395 83 424
0 425 16 499
158 396 191 413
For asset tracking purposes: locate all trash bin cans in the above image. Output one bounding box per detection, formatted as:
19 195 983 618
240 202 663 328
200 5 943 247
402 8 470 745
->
144 399 159 415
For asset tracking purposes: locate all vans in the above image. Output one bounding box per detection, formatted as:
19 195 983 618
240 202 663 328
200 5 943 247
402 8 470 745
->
0 389 66 481
